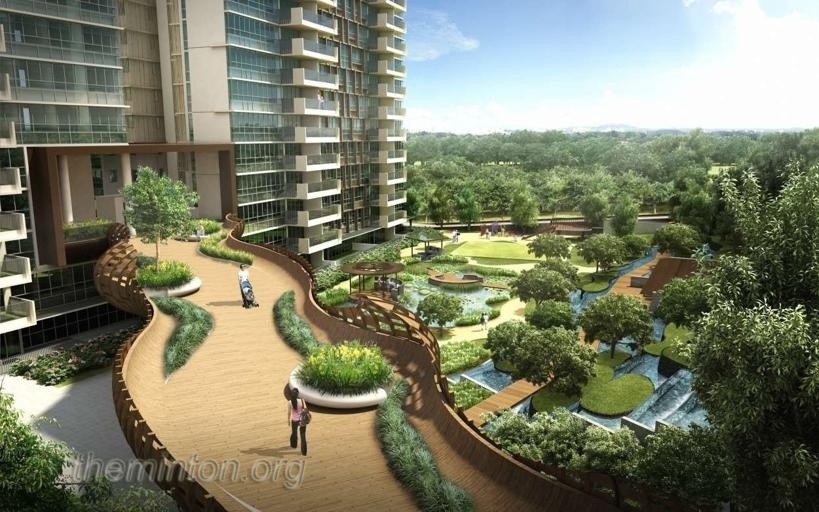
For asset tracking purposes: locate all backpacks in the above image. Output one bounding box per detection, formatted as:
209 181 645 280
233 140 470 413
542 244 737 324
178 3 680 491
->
300 399 312 426
